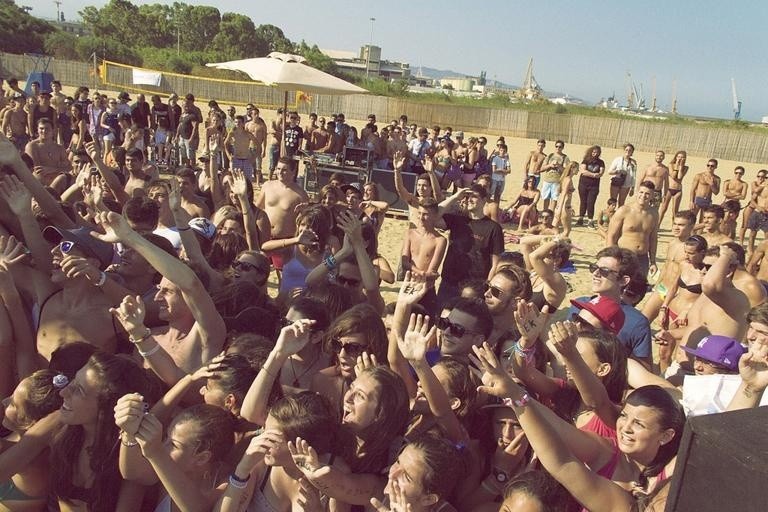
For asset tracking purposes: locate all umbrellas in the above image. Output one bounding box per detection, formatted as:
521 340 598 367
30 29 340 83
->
206 51 369 157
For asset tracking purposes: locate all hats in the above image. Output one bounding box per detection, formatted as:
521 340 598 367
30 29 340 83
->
480 383 538 410
91 168 100 174
12 94 25 101
47 341 99 379
569 294 626 336
39 91 52 97
437 135 450 141
340 182 366 197
419 128 430 137
189 218 217 244
455 132 465 137
678 333 743 367
117 92 132 102
278 108 285 113
221 308 281 339
40 223 116 264
113 112 131 121
198 151 211 161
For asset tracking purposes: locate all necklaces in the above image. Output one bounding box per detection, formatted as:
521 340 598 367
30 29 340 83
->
290 355 321 388
531 275 544 290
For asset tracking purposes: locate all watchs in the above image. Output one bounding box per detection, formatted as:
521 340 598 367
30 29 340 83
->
490 469 510 484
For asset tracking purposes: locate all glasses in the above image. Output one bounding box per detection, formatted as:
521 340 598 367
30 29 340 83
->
64 102 71 105
529 181 535 184
337 119 342 122
334 273 363 286
735 171 742 176
110 101 117 104
764 176 768 178
480 282 501 299
82 91 89 95
439 141 445 143
93 97 102 100
587 262 619 277
320 120 324 122
477 141 483 144
571 312 598 339
48 238 74 252
707 165 715 168
410 127 416 130
757 175 763 178
496 144 502 147
329 340 365 359
447 132 451 136
555 145 561 148
290 116 297 120
228 109 234 111
231 257 264 276
436 317 464 339
693 356 715 369
541 215 549 220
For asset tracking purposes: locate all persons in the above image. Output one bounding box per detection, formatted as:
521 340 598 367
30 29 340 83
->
475 266 548 376
0 77 267 512
746 172 768 256
526 209 559 237
459 388 538 512
267 198 505 511
745 235 768 287
466 340 674 511
576 145 605 230
638 210 695 322
596 197 617 239
622 275 647 307
519 235 574 313
540 140 571 214
656 151 689 232
608 144 637 208
487 469 582 512
553 160 581 238
565 247 654 371
738 302 768 353
515 296 680 405
491 249 526 273
723 166 748 202
638 151 669 220
471 344 768 512
522 140 546 191
491 326 546 395
267 104 512 199
643 329 744 384
721 201 741 241
718 245 768 312
739 169 766 247
689 159 722 228
693 206 731 267
504 177 545 231
653 235 713 372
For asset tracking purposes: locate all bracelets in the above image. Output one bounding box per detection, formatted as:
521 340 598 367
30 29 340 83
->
323 260 333 271
658 304 670 314
330 254 337 264
95 272 105 287
500 391 530 410
139 345 160 356
673 169 678 171
232 473 251 482
327 257 335 268
177 226 190 231
128 328 152 343
121 432 138 447
479 475 504 500
282 239 287 249
229 475 248 489
511 340 538 365
